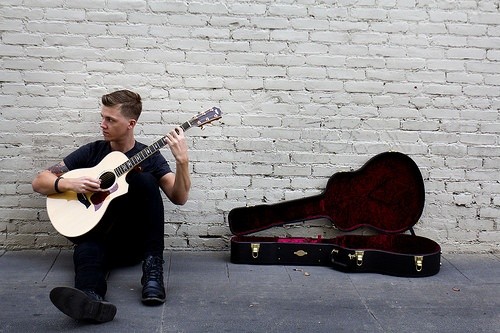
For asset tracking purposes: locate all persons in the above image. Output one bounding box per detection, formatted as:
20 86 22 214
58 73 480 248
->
32 90 191 323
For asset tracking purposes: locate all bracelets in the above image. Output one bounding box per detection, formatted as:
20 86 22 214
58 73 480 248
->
55 177 64 193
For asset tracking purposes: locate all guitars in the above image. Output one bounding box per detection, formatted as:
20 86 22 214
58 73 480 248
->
46 106 224 245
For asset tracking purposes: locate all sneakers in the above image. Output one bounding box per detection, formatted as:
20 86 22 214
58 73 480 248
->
140 255 166 304
48 286 116 321
